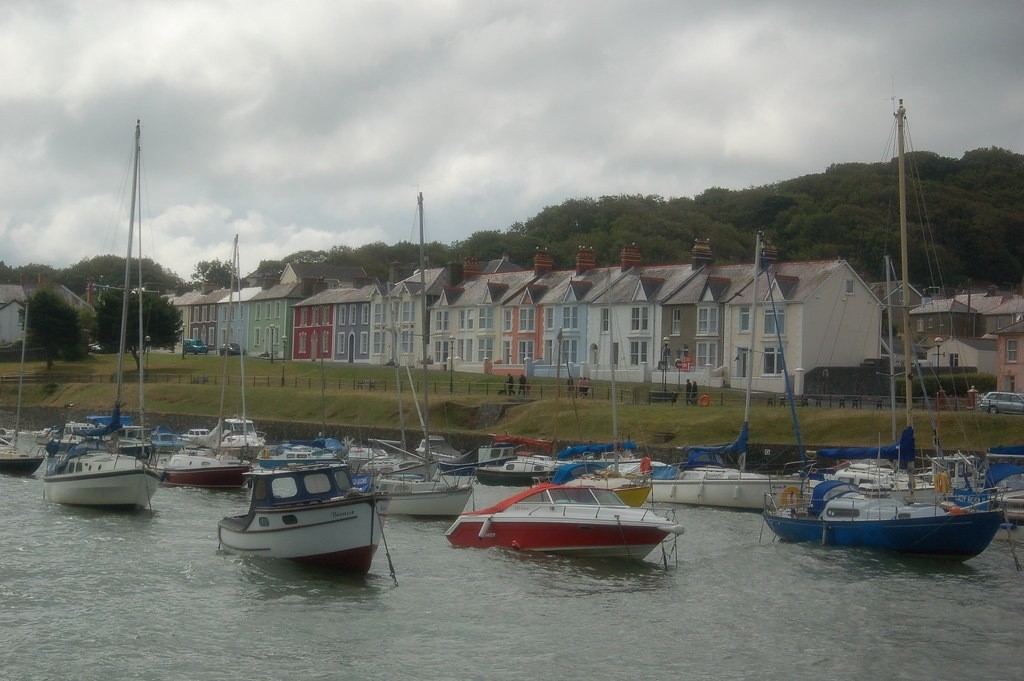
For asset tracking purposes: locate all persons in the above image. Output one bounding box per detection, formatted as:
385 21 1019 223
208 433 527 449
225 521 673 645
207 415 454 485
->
582 377 589 399
567 376 574 397
686 379 692 406
526 382 532 396
690 381 698 406
578 377 587 399
518 372 526 396
504 372 515 396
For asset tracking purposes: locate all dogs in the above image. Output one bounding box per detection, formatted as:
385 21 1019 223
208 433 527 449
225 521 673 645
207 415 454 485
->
497 388 498 395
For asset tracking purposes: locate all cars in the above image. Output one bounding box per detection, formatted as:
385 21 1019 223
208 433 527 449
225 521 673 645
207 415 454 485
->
218 342 248 357
88 343 105 354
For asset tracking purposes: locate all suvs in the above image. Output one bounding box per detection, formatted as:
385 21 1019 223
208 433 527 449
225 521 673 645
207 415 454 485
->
183 338 209 355
980 391 1024 416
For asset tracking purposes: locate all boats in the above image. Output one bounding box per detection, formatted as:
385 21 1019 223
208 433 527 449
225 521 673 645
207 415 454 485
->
44 190 1024 534
216 460 393 578
443 453 687 563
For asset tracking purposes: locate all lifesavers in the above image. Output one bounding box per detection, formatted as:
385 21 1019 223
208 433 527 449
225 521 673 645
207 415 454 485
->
701 395 710 406
780 487 809 508
262 449 269 460
934 473 949 495
639 457 650 473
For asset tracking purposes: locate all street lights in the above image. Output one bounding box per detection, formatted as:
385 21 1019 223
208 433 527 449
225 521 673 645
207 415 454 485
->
281 335 288 387
145 335 152 383
449 335 455 394
663 336 672 392
270 323 276 363
935 336 944 412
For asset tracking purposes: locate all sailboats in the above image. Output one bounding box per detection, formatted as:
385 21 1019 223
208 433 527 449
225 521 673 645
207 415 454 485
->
158 233 255 488
42 116 164 513
0 300 45 478
754 96 1014 561
